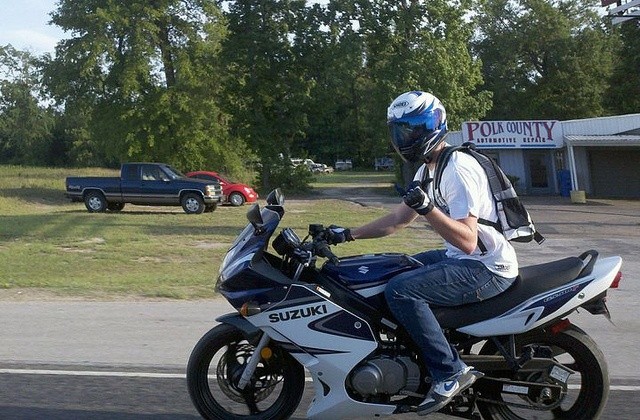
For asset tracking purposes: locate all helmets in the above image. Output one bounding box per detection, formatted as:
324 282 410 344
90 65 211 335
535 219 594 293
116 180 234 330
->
387 91 449 163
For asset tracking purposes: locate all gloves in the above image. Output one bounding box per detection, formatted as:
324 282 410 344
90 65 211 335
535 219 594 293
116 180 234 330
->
403 186 434 215
325 224 355 246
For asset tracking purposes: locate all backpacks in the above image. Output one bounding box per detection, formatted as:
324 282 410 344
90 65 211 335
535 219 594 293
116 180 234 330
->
421 142 545 256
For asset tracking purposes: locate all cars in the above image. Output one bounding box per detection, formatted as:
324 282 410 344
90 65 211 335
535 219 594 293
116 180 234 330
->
186 171 258 206
323 164 333 174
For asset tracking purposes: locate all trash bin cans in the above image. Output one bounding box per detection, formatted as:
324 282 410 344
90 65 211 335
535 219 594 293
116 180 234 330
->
559 170 570 195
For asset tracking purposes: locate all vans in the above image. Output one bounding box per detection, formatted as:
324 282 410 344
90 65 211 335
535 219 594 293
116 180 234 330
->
292 158 323 172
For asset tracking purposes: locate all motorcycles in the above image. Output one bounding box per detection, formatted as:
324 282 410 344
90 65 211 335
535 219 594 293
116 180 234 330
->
186 189 621 419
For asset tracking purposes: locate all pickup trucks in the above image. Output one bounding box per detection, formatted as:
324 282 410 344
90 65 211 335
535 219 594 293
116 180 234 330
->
66 163 221 213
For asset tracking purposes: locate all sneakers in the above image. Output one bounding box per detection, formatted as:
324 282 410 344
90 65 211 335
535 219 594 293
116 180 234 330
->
416 366 477 416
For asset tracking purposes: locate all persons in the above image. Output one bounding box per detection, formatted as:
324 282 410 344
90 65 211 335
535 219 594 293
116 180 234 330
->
328 90 520 417
